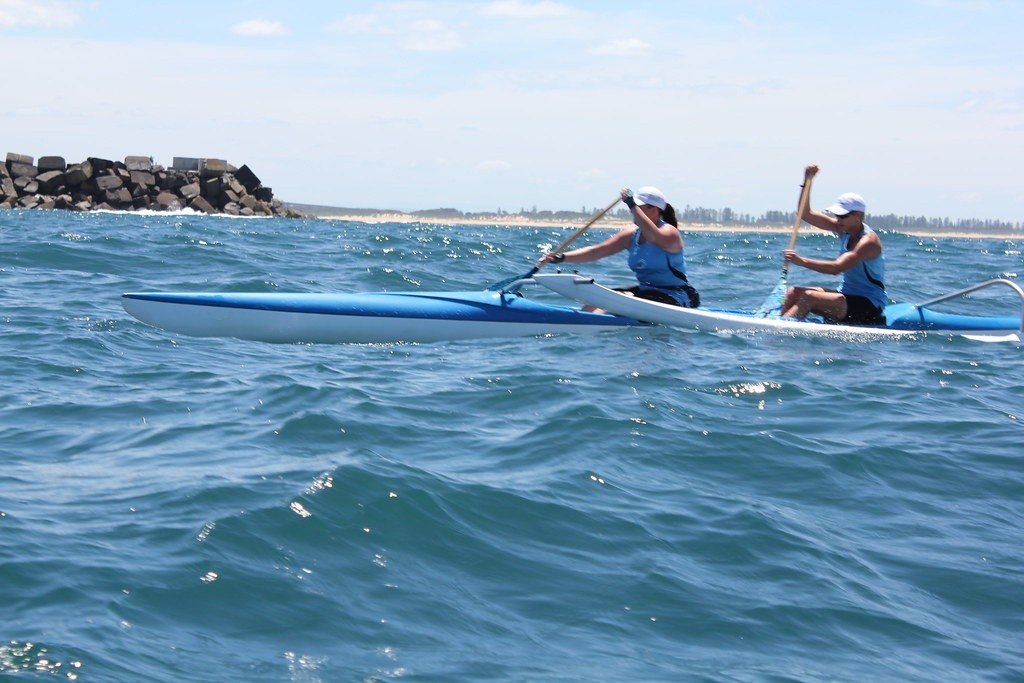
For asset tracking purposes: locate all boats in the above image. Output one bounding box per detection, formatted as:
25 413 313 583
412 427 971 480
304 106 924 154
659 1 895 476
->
121 271 802 345
534 272 1024 349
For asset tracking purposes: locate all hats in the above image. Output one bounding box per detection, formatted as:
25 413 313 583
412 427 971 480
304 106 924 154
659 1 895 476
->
632 186 666 210
825 192 866 215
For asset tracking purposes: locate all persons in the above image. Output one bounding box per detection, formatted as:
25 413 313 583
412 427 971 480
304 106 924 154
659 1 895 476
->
539 187 692 313
781 163 886 322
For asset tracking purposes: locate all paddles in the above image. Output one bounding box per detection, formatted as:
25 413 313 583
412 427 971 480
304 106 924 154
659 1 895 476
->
756 171 814 319
481 196 624 292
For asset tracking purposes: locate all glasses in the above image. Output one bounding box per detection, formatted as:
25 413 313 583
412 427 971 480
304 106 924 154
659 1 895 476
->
836 210 856 219
